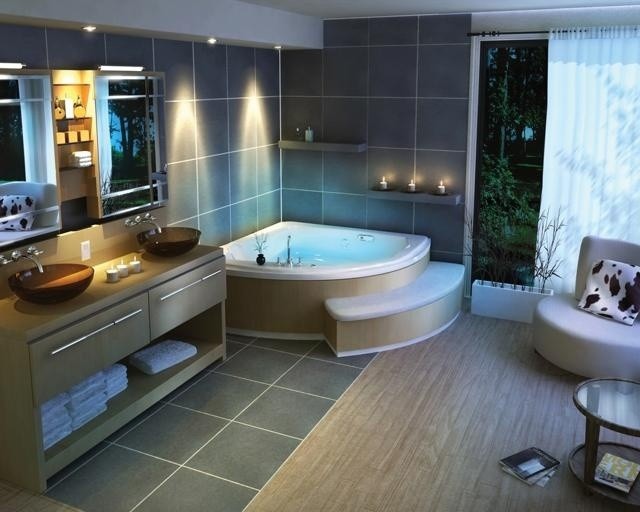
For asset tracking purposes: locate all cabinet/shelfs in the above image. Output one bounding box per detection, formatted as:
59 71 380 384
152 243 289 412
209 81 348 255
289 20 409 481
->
0 256 229 494
50 67 95 173
276 138 460 206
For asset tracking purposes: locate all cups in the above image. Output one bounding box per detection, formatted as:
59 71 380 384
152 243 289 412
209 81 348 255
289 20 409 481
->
305 129 314 142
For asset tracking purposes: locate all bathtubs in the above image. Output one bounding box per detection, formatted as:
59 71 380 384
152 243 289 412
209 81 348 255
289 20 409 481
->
223 220 430 279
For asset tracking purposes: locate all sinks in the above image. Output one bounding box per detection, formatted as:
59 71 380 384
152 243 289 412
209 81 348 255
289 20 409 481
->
137 227 202 256
7 263 94 305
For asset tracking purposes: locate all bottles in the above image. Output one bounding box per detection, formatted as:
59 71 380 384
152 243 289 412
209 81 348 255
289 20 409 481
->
54 95 87 119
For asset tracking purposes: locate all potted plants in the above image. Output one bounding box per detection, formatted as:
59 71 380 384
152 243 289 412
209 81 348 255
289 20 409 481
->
466 153 565 324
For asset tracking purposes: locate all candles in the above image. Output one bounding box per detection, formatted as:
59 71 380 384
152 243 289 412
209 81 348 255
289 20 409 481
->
438 180 446 194
407 179 416 191
378 175 388 191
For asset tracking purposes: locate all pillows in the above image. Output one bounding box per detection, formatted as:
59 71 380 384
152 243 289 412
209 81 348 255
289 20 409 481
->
576 257 638 326
0 195 36 231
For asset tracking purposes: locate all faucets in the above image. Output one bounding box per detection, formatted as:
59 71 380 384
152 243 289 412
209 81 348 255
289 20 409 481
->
14 251 44 274
125 216 162 235
288 236 291 263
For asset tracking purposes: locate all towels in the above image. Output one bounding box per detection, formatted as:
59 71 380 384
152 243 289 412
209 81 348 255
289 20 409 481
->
40 338 198 452
69 150 92 169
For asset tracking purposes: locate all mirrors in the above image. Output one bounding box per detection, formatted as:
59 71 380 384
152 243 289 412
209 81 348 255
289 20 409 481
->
0 69 64 250
86 69 170 220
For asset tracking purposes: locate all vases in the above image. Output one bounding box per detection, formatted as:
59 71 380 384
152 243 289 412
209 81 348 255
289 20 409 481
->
256 254 266 266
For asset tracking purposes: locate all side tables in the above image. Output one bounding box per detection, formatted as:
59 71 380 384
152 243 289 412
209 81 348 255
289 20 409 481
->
564 376 640 507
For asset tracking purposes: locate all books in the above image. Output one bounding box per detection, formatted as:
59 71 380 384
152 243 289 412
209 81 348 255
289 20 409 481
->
501 462 559 486
498 445 561 480
533 464 560 488
594 452 640 494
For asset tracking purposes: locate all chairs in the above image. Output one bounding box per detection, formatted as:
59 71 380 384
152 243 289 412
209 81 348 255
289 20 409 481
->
532 235 640 384
1 181 59 244
